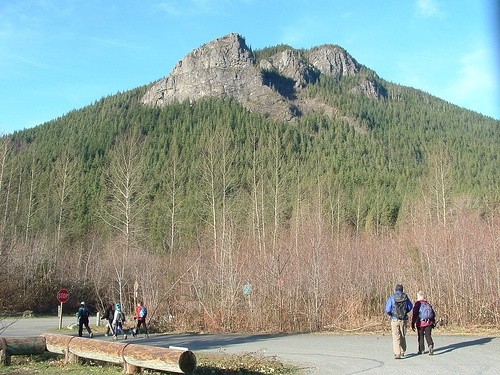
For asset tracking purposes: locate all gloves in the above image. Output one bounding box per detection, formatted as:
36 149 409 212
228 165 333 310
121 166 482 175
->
412 324 416 331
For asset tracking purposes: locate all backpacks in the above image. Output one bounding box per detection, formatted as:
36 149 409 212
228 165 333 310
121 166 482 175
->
416 300 435 322
393 293 408 320
139 306 147 318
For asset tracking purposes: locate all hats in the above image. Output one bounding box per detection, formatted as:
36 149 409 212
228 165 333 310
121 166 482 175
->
116 303 121 309
417 291 425 299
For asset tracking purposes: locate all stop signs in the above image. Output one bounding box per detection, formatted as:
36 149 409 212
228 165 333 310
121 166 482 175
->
57 290 70 304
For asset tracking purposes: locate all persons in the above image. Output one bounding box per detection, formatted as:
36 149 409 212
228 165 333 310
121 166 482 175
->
100 304 116 338
132 301 149 337
77 302 93 338
411 290 436 355
112 304 128 340
385 285 413 359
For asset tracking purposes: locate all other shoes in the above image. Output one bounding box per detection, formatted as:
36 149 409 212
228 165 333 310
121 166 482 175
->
400 353 405 358
429 345 434 355
418 351 424 355
90 333 93 338
393 354 400 359
105 333 149 340
